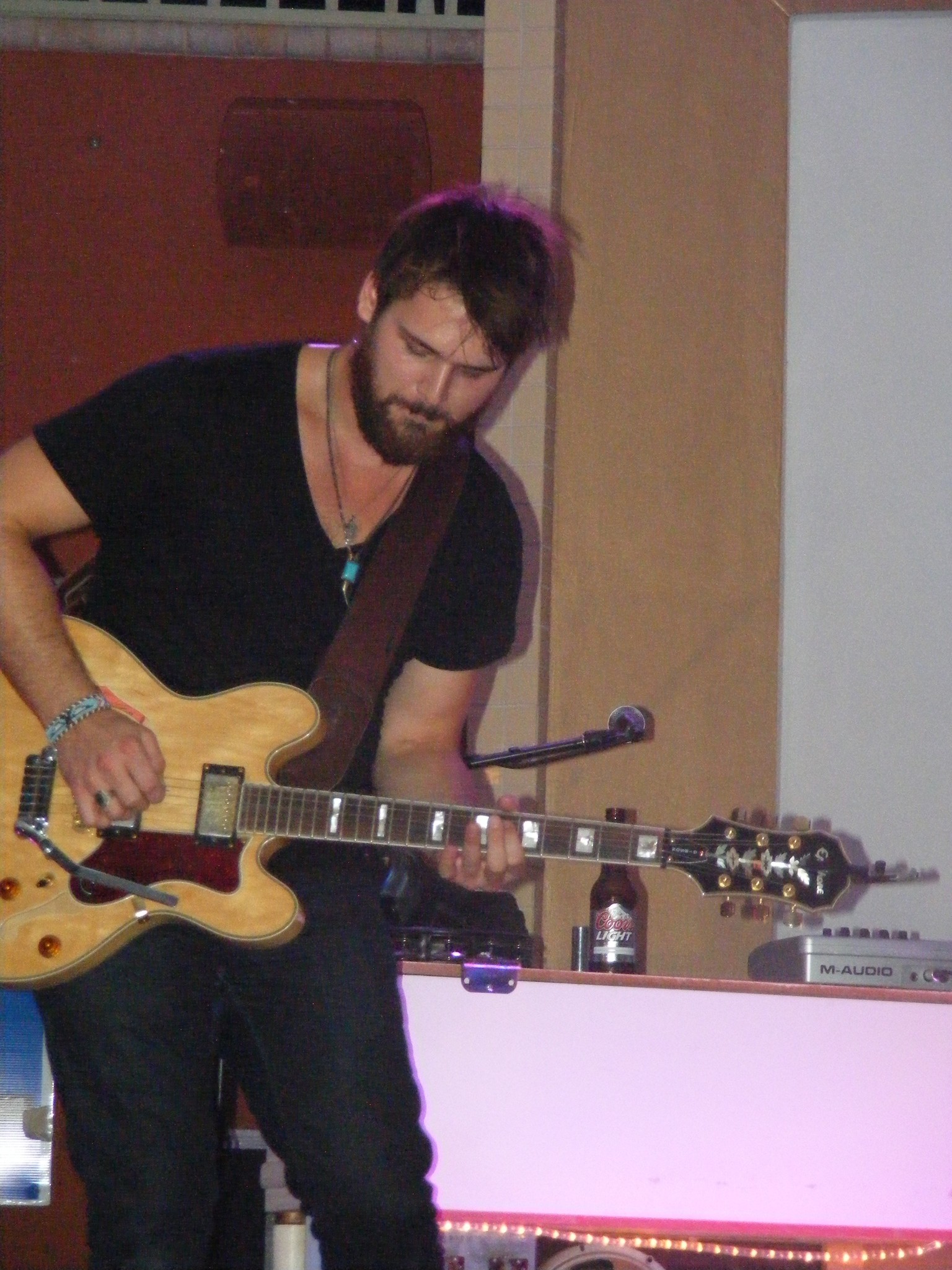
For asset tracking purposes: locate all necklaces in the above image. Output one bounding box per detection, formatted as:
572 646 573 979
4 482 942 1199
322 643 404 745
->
325 343 418 607
330 353 404 544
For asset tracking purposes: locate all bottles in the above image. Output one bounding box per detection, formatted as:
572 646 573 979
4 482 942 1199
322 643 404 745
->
586 806 640 977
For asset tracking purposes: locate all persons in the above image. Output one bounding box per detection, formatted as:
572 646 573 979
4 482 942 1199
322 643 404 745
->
3 185 582 1270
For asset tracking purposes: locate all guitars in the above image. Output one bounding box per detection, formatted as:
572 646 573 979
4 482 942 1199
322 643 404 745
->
1 609 939 991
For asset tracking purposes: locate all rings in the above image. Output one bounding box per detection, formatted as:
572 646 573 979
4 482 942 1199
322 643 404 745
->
95 789 115 807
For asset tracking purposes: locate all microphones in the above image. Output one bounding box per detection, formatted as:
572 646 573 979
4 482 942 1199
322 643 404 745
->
608 705 647 745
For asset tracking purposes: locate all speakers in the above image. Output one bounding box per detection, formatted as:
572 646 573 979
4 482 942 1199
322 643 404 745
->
216 93 436 256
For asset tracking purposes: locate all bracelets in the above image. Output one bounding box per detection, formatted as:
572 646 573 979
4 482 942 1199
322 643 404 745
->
45 691 109 745
